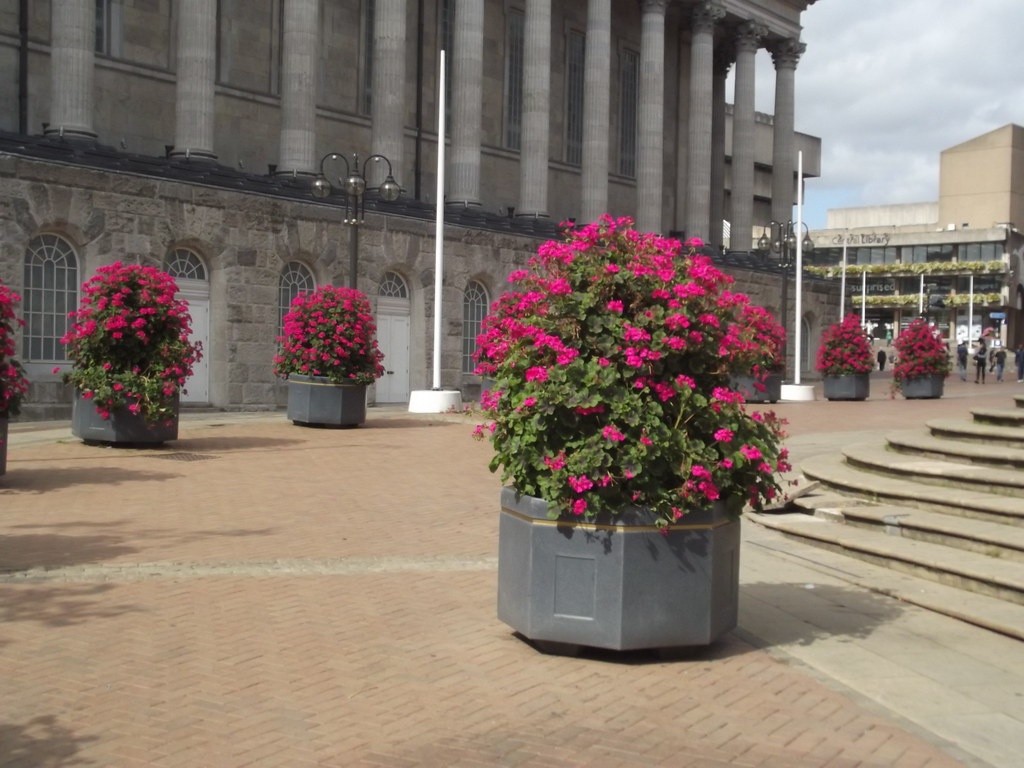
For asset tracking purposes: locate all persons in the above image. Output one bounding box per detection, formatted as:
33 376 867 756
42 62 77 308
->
988 348 996 372
994 347 1006 381
1015 344 1024 383
867 320 891 372
957 341 968 381
973 343 988 384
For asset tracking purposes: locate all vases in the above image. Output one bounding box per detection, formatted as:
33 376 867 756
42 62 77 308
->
69 385 179 446
495 484 745 655
824 373 869 402
897 374 945 401
286 372 368 428
0 416 9 476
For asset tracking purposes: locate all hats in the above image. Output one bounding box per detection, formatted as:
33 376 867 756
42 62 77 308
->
977 338 984 341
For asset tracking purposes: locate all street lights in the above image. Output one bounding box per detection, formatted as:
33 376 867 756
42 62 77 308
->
309 148 406 289
757 218 815 381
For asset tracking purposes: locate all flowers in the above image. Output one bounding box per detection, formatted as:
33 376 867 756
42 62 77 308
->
891 317 954 397
460 214 798 541
815 314 876 374
0 279 31 415
52 261 205 430
272 285 385 386
